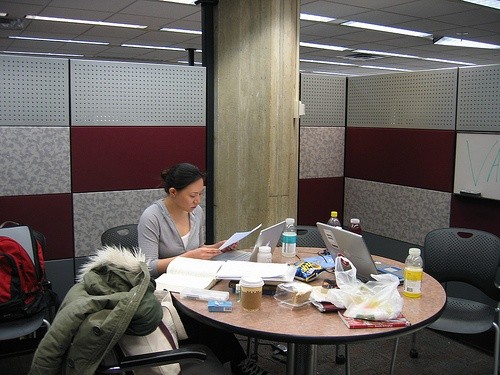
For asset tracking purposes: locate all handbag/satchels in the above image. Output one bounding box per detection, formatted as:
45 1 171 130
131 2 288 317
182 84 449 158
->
335 256 404 322
116 290 188 375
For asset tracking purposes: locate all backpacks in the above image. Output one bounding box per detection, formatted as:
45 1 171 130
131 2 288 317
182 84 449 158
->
0 221 57 321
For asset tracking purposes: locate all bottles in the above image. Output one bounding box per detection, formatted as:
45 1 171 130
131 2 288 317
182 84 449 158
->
282 217 297 258
327 211 342 229
257 246 272 263
348 218 361 235
402 248 423 298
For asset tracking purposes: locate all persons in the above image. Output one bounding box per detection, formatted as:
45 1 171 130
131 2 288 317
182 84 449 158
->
138 164 271 375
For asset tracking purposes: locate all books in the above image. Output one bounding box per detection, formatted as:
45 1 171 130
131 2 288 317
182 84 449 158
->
154 257 225 294
338 310 412 329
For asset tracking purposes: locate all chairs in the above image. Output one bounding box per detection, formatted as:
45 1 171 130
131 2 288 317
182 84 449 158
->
253 225 346 367
0 221 228 375
410 227 500 375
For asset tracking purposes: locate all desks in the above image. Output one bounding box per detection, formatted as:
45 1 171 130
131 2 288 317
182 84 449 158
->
170 247 447 375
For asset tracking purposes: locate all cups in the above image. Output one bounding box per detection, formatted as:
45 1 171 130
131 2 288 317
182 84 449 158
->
239 274 264 312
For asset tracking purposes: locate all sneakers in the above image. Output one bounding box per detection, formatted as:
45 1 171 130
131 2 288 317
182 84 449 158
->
230 357 272 375
